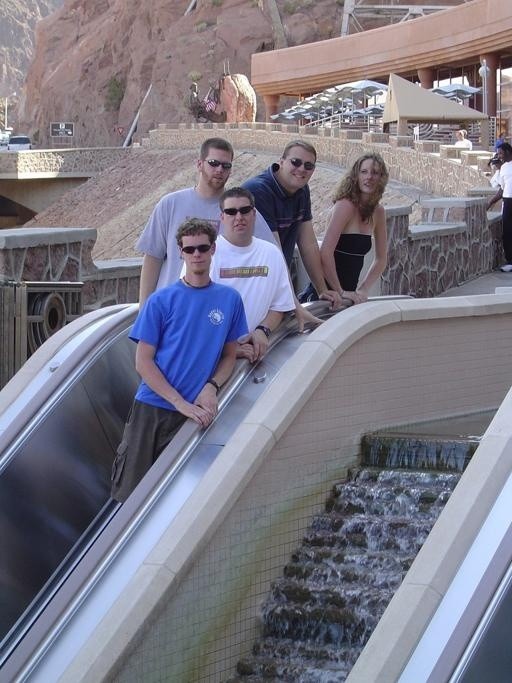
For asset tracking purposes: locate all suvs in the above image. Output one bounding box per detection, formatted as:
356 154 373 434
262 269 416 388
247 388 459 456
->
7 134 32 150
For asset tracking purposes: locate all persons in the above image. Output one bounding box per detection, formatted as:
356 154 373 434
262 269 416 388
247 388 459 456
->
180 186 297 364
486 157 503 211
487 142 512 272
455 130 473 153
188 82 226 121
241 140 342 335
136 136 352 332
109 218 249 503
302 148 389 304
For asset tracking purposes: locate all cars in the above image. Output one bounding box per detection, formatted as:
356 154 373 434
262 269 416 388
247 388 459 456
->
0 132 9 145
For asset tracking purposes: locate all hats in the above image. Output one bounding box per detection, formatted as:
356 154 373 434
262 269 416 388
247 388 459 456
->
491 152 501 166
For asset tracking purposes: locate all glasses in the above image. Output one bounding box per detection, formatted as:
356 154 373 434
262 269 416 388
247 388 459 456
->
223 206 253 215
180 245 211 254
285 157 315 171
204 159 232 169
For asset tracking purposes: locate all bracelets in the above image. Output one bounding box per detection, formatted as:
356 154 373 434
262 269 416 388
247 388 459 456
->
340 290 344 296
209 379 220 391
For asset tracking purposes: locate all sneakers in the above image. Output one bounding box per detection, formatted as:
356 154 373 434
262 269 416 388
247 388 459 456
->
499 264 511 273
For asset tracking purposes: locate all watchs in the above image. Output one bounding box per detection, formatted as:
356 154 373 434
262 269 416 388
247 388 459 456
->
255 327 275 338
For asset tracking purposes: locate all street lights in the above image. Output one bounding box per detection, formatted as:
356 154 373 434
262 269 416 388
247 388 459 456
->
476 58 490 114
5 91 17 129
